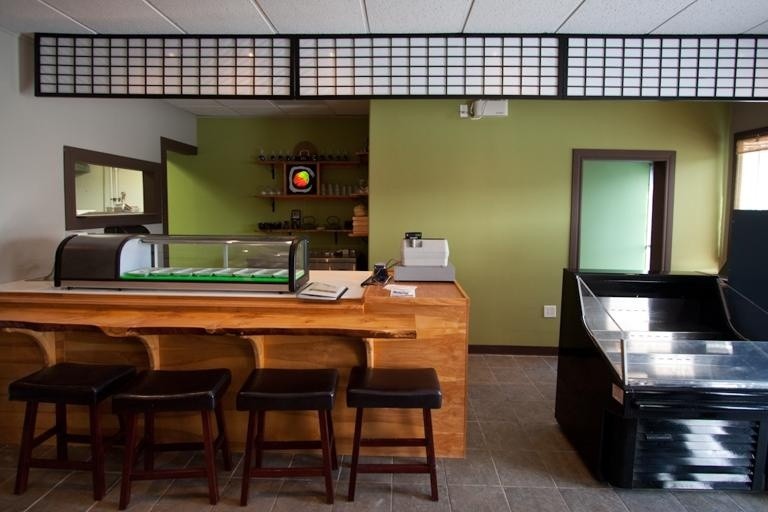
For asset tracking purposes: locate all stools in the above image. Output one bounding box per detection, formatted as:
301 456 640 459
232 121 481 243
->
236 368 339 506
346 365 442 501
8 362 139 500
111 367 233 510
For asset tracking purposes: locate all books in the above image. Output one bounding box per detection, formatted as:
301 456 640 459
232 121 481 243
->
295 282 348 301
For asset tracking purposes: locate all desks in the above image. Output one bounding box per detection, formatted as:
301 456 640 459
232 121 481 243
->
0 306 418 371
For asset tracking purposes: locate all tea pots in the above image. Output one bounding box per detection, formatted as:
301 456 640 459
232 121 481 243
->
326 216 341 229
301 216 319 229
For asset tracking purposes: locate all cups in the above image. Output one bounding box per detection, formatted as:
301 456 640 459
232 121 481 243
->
321 183 352 196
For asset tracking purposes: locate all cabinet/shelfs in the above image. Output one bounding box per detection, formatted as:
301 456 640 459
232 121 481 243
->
255 160 362 233
348 152 368 237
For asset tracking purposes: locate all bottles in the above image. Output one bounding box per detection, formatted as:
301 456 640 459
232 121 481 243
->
258 147 348 161
